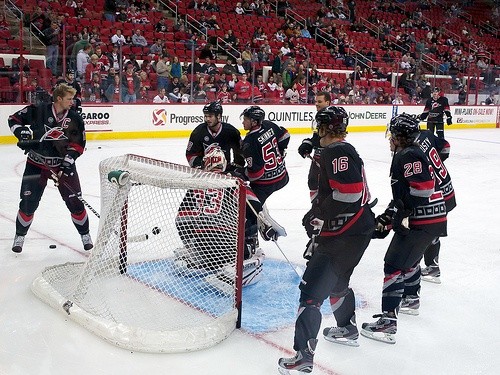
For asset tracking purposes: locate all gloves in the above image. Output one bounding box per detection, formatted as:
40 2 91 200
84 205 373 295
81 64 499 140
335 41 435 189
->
17 128 34 155
375 202 400 233
446 118 452 126
417 113 428 121
302 209 322 238
54 154 75 177
193 157 204 170
298 138 314 159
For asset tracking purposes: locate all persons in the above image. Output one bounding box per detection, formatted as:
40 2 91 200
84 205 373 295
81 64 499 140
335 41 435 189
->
8 82 93 259
184 100 243 170
0 0 500 107
417 130 458 283
298 92 331 205
277 106 377 375
173 151 287 293
421 87 453 140
359 113 447 344
240 104 290 202
54 69 82 114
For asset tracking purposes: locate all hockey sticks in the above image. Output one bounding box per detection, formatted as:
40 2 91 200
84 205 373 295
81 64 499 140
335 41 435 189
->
32 155 150 243
417 120 448 124
307 152 379 208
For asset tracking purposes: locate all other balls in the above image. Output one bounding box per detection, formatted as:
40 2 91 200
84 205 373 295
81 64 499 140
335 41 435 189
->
49 244 57 249
97 146 101 149
152 227 161 236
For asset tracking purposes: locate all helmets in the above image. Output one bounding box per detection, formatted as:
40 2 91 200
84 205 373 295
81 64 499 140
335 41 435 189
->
202 101 224 115
389 112 420 135
239 106 266 126
431 86 440 94
65 69 75 81
315 106 350 134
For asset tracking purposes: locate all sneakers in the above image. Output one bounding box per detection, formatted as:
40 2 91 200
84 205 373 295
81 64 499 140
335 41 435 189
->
80 232 93 254
11 234 26 258
276 338 319 375
323 314 361 348
397 285 422 316
420 255 441 284
359 305 401 344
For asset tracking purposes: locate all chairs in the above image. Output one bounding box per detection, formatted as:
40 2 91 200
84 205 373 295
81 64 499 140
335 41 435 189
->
0 0 500 106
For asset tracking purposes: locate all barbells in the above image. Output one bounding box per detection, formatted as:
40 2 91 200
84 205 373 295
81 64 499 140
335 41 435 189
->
246 198 308 285
